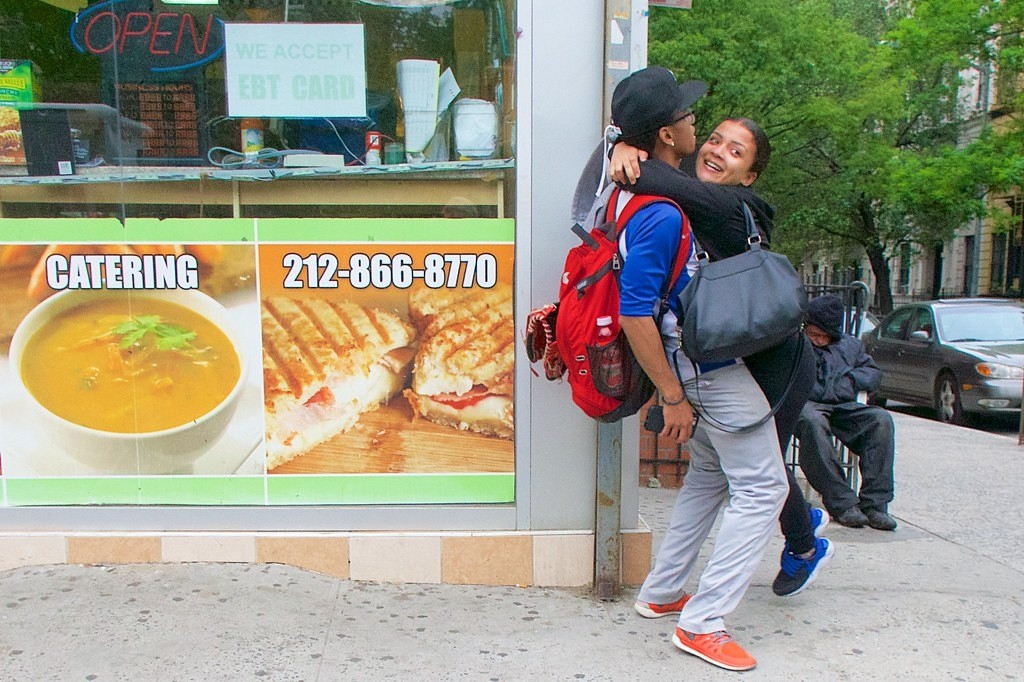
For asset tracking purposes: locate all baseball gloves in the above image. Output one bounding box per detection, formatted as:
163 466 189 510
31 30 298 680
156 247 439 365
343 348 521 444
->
523 299 565 384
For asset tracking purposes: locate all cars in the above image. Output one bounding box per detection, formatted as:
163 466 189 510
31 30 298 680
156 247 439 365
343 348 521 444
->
843 304 880 339
861 297 1024 427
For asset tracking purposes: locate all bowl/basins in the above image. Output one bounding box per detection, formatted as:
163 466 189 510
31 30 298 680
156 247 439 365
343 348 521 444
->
7 276 252 474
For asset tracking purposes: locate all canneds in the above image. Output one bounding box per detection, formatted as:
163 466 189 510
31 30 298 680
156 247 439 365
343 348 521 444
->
364 130 382 167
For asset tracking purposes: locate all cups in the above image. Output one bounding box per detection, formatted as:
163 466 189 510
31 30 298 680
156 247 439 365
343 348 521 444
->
384 142 404 164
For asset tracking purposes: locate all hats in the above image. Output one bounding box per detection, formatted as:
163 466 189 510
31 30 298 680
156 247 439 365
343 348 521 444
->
609 66 707 138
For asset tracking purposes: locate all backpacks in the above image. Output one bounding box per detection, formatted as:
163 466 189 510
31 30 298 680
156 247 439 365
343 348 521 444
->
554 187 691 424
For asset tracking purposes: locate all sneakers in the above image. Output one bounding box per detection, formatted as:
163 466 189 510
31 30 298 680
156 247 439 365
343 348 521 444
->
779 507 830 567
772 535 834 596
672 626 757 670
634 592 692 618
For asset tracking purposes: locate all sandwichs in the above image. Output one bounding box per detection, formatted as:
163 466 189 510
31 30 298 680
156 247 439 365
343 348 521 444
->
261 285 516 471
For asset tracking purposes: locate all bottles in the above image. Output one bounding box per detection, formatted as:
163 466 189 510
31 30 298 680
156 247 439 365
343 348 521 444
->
240 117 265 153
594 315 626 397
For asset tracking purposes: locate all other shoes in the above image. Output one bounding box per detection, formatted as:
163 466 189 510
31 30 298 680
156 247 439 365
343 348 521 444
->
836 505 867 528
862 507 897 531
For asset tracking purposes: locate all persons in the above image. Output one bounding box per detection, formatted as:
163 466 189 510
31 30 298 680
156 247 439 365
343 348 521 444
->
608 117 835 597
604 64 790 670
795 296 897 529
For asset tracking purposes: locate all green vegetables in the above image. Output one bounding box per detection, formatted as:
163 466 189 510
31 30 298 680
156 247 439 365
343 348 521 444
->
113 314 197 351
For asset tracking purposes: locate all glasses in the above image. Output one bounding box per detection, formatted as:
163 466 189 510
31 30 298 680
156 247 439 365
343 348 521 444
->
663 105 695 130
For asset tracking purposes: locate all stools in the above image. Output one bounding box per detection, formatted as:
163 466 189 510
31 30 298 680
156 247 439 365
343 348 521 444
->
786 434 860 509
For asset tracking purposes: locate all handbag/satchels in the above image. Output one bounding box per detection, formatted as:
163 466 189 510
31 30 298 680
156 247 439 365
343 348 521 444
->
674 200 810 434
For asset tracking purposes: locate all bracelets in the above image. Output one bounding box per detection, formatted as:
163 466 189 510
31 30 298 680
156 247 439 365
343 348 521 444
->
662 389 685 404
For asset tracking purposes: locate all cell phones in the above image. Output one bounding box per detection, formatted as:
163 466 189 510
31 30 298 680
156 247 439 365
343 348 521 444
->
643 406 699 439
